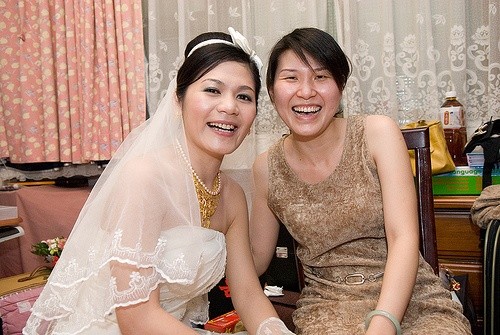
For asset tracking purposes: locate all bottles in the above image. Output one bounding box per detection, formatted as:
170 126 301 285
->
440 91 468 166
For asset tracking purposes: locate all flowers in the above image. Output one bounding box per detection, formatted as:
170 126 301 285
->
29 235 67 272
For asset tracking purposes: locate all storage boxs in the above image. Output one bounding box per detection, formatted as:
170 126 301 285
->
0 206 18 220
432 166 500 197
204 307 245 333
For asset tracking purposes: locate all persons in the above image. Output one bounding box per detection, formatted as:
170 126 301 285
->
23 32 295 335
250 28 472 335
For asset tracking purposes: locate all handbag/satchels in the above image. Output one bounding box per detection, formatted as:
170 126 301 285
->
440 261 480 335
400 120 456 177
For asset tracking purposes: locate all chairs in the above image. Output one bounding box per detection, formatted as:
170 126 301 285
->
271 127 440 332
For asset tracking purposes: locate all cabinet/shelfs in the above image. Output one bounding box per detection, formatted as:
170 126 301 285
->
433 199 483 335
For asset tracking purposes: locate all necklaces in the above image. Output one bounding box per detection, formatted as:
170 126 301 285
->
181 155 221 228
176 138 221 195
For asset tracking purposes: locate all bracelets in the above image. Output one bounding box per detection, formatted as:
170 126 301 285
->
365 309 401 335
